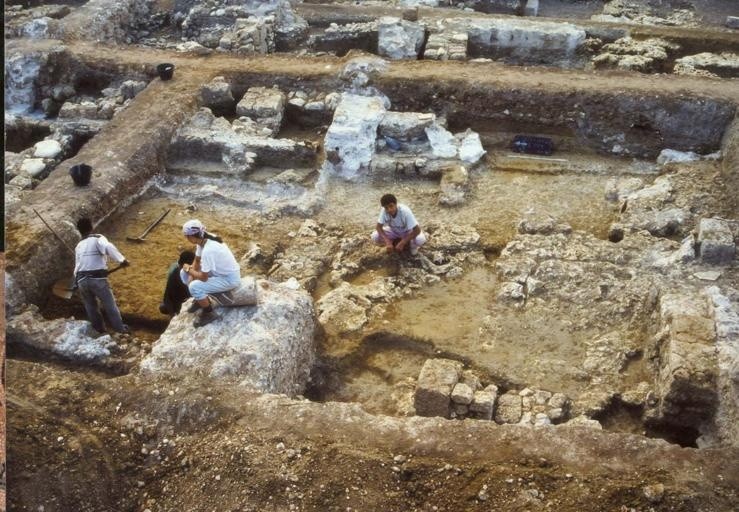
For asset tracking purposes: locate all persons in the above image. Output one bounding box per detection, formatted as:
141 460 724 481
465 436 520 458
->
180 220 241 328
160 251 195 315
71 217 129 332
370 195 426 257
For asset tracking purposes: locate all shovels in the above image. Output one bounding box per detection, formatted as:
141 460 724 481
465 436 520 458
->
53 262 128 300
127 208 172 241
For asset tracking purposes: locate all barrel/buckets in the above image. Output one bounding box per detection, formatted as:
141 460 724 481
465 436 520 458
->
68 163 92 186
157 63 175 81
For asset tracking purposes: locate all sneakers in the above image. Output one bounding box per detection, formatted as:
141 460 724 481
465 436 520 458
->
194 310 218 327
187 299 210 313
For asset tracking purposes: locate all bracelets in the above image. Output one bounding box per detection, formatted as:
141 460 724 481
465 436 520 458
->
185 265 192 273
